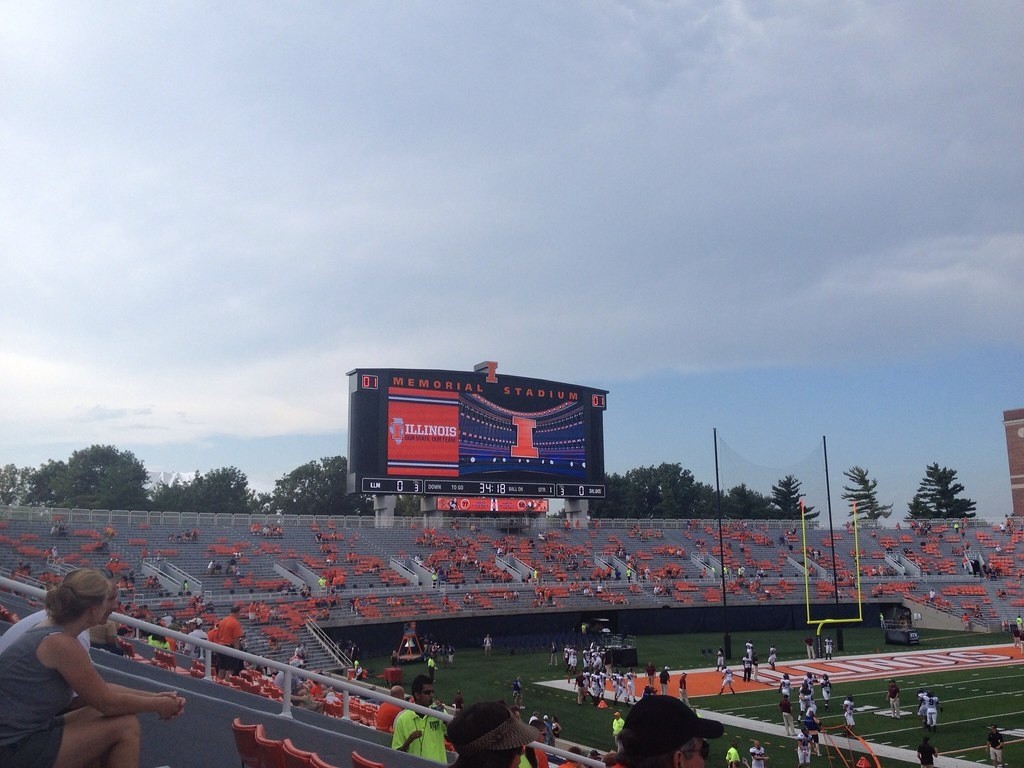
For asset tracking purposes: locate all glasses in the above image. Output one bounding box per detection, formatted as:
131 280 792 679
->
671 740 710 760
420 689 434 695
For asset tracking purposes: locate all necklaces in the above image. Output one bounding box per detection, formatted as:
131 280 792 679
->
411 717 429 757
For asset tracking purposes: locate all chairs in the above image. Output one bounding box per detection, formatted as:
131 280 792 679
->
0 605 456 768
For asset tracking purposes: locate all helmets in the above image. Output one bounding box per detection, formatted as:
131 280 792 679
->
783 671 936 703
583 664 634 677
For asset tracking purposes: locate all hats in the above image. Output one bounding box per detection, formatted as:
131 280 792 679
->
923 736 930 742
453 705 540 757
543 715 548 720
682 673 687 675
621 694 724 758
665 666 670 671
991 723 998 729
613 712 621 717
528 715 538 724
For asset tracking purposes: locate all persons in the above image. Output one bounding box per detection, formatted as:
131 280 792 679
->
923 690 943 733
820 673 831 712
718 665 735 695
886 680 900 719
824 636 832 660
749 740 769 768
678 673 689 706
659 666 670 695
778 694 798 736
0 504 1024 768
716 647 725 671
842 694 855 736
803 636 816 660
